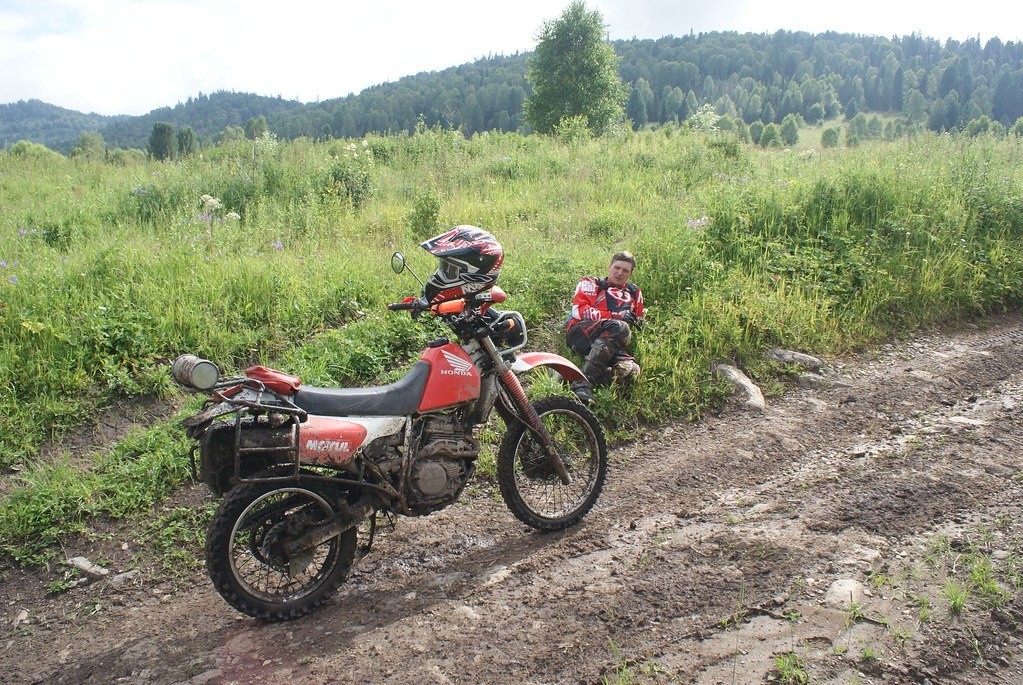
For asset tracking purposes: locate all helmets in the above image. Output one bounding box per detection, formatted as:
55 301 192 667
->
420 225 504 304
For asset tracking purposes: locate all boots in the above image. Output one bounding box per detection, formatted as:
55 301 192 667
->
570 337 619 403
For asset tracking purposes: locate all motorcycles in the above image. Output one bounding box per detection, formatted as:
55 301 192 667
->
176 249 609 625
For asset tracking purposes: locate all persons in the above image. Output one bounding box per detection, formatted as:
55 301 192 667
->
564 251 643 409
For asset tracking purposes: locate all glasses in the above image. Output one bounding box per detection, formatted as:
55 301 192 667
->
439 257 464 282
